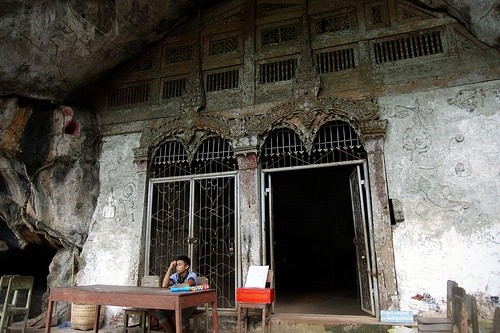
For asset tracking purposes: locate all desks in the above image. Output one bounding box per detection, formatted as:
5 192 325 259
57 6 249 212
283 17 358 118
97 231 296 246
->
45 284 219 333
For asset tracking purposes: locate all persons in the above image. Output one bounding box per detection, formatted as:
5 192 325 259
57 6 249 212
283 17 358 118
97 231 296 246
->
157 256 199 333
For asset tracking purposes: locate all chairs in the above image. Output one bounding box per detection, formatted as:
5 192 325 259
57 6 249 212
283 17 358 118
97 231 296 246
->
183 277 209 333
466 295 479 333
122 275 161 333
417 286 466 333
0 275 34 333
237 269 275 333
0 275 21 333
416 279 459 325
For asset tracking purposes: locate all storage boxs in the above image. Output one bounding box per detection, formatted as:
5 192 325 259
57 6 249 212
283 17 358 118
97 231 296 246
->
236 287 275 304
70 302 105 331
